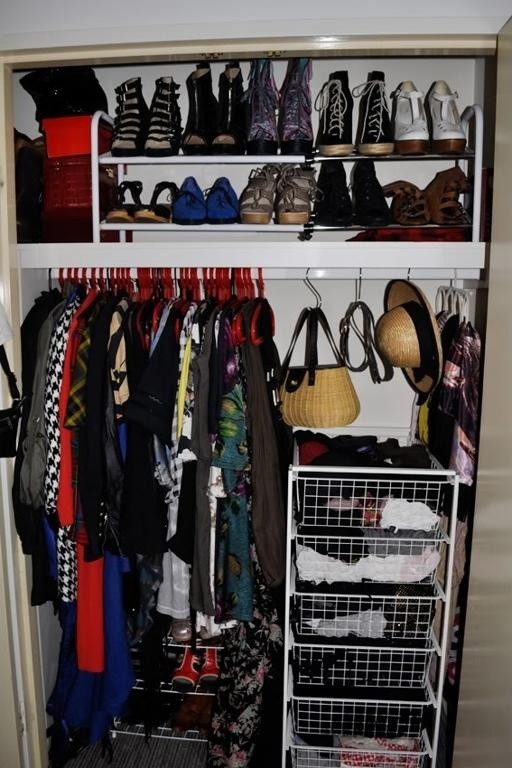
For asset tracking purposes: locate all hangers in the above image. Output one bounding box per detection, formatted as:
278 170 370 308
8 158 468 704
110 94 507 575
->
48 266 277 347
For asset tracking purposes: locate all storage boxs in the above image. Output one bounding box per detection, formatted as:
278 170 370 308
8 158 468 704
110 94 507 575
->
39 115 116 158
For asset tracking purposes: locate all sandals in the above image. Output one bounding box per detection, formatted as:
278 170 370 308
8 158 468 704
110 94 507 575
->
105 181 178 224
395 79 466 155
110 76 183 157
240 164 324 225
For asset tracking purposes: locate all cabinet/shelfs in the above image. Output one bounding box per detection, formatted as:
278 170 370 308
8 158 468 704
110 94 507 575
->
1 15 512 767
282 433 460 767
83 106 483 244
109 619 224 744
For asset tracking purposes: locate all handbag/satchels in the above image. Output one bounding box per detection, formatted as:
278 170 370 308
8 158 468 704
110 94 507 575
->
19 67 108 121
277 307 361 429
41 156 116 221
1 406 21 458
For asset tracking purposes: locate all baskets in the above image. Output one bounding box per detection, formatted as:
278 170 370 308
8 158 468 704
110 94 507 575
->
292 436 450 767
292 436 450 768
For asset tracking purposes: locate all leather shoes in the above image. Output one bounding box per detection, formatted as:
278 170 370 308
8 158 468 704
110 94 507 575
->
172 618 194 642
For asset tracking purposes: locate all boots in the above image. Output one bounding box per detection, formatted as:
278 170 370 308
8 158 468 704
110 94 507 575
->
181 58 394 156
383 166 471 225
316 160 390 227
173 694 212 732
170 638 223 689
173 176 241 225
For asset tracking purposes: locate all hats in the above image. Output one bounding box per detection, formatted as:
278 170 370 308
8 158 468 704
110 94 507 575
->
376 279 444 395
